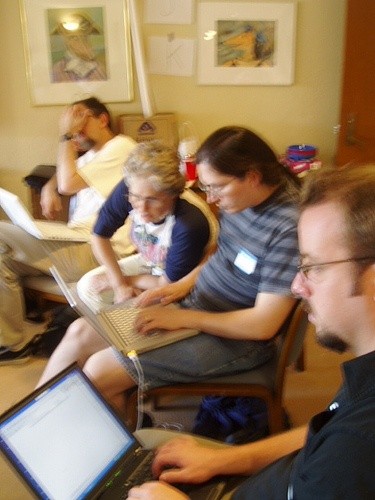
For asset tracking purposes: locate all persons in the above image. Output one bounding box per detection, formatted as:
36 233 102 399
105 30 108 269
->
48 141 211 347
126 167 375 499
36 127 302 433
0 98 140 364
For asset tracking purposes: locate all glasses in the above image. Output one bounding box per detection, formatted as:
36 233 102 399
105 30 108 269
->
298 256 374 279
127 193 174 207
198 174 240 193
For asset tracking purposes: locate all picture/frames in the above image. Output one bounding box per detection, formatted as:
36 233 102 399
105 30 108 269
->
195 0 299 87
18 0 134 106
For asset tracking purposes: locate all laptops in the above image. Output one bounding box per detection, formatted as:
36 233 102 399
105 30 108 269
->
0 188 90 243
48 264 200 358
0 360 230 500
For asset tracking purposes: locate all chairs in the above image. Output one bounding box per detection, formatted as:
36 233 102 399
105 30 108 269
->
128 293 308 438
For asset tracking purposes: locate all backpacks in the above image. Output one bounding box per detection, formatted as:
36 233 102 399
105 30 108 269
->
33 303 80 357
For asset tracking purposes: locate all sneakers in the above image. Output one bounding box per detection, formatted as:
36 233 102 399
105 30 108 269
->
0 344 31 366
